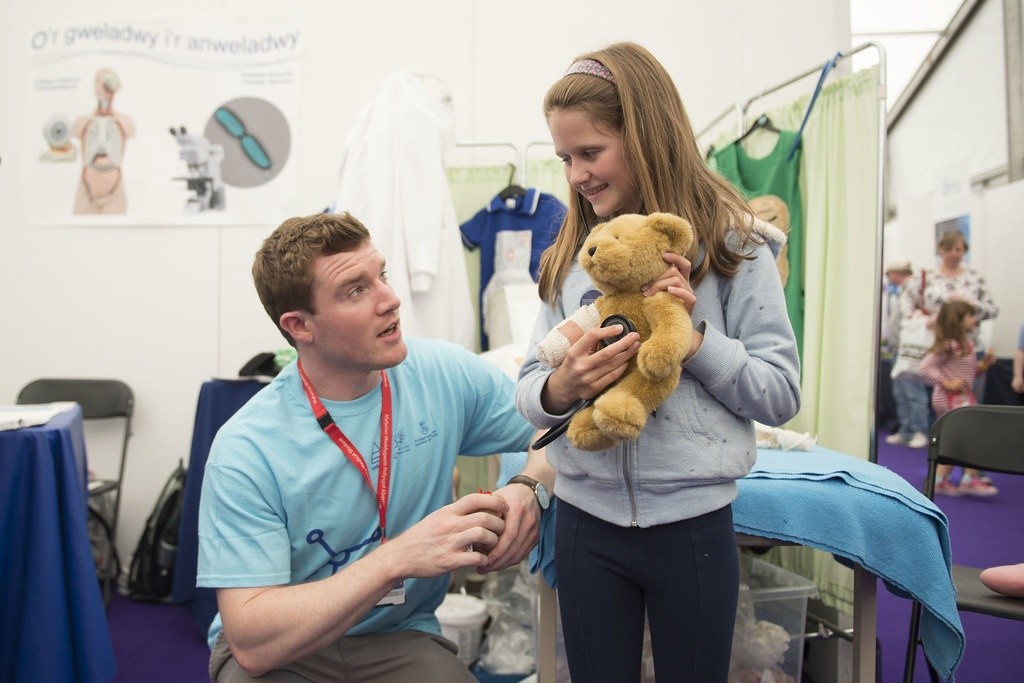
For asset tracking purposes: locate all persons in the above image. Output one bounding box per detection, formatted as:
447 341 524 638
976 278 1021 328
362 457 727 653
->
909 230 999 405
197 214 556 683
916 300 998 497
514 42 801 683
1012 320 1024 392
886 257 930 448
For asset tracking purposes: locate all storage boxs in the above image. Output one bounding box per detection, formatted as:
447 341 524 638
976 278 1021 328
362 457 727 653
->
533 550 817 682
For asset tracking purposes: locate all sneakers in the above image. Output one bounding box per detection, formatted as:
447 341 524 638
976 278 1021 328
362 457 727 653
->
934 480 960 497
907 432 930 448
885 432 907 444
958 474 999 497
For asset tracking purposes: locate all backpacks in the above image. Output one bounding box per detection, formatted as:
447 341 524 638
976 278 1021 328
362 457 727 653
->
126 458 186 605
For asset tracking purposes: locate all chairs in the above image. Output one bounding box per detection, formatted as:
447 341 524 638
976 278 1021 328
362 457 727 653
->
16 378 133 611
904 403 1024 683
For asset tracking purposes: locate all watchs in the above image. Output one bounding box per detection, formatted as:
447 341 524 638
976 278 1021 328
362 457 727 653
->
507 475 549 516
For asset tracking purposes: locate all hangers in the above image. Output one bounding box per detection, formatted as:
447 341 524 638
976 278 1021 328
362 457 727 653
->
500 162 528 200
735 93 783 144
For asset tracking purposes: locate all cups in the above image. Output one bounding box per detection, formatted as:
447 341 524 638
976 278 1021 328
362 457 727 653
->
433 592 488 667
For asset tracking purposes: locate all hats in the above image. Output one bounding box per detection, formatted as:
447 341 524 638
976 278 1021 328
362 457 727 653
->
887 260 912 272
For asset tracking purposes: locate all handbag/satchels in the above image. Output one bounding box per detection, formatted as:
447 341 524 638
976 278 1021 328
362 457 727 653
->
891 310 936 379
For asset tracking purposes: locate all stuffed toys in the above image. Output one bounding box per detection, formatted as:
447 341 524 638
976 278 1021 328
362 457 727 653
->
535 213 693 452
